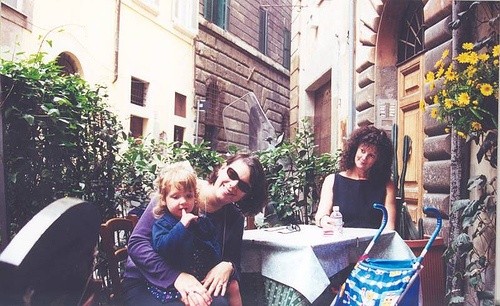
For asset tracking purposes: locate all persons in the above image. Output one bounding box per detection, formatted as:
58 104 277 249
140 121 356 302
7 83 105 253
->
313 126 396 293
143 160 243 305
122 151 269 306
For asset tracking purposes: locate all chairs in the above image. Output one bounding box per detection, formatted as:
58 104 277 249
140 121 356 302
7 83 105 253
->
99 214 140 306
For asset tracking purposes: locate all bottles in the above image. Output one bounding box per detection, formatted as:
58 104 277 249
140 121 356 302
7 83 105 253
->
330 206 343 236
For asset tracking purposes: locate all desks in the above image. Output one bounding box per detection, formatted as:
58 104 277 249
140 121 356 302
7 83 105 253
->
241 225 416 306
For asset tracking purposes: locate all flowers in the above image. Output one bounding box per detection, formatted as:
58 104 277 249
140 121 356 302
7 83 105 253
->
420 42 500 143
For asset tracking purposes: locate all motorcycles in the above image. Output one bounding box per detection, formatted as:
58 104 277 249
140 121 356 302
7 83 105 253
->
222 92 303 228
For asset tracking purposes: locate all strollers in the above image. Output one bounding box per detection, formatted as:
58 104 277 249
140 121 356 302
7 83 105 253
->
330 203 443 306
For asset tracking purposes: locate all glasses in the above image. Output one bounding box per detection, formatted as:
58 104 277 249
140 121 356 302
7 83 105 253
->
264 222 302 234
225 167 252 195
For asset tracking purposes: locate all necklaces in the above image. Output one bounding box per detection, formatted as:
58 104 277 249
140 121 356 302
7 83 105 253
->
204 183 227 258
